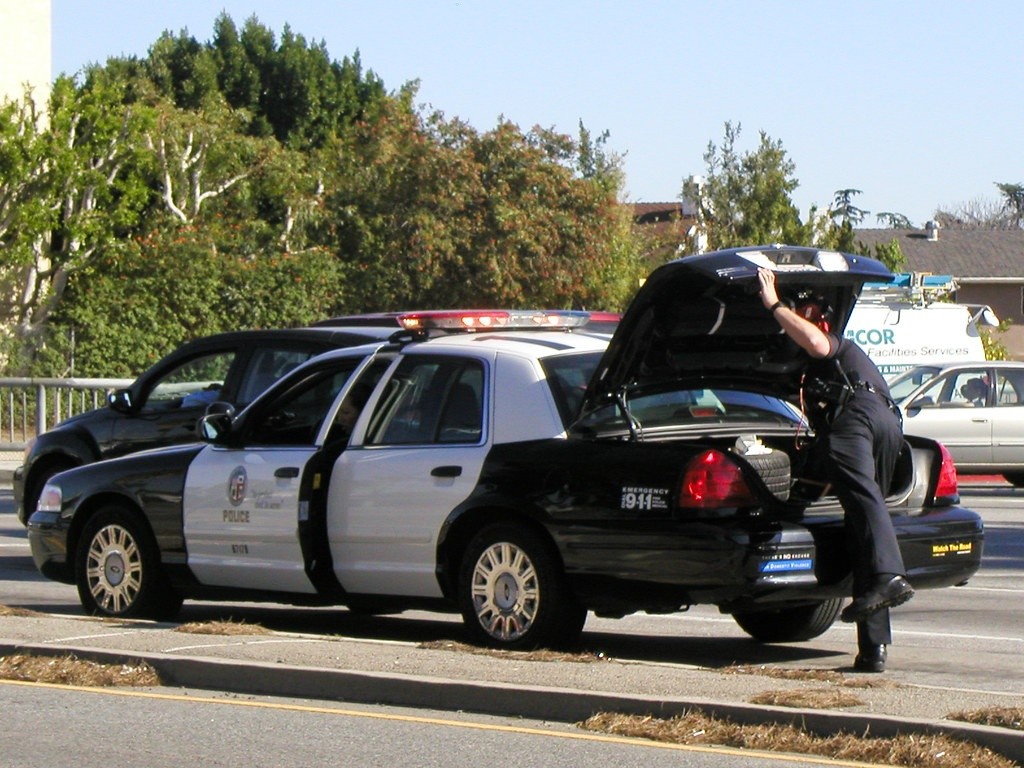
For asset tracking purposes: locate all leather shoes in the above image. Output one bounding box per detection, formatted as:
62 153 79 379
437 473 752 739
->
854 643 888 674
841 576 916 622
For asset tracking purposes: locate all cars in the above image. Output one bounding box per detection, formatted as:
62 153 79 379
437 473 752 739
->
11 308 624 531
26 241 990 654
886 360 1020 489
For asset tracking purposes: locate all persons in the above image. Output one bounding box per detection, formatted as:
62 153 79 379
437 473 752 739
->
335 385 372 437
753 264 916 673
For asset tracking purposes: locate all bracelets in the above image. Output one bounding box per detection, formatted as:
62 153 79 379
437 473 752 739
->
770 301 785 316
